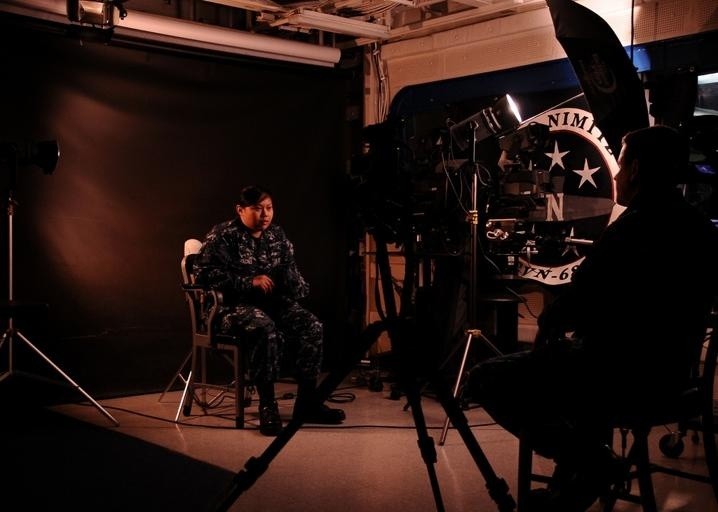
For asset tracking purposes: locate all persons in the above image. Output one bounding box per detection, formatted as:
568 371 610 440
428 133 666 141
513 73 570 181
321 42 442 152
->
188 183 348 438
464 124 718 510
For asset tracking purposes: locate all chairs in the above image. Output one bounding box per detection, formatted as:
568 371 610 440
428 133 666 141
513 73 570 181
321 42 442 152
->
181 238 281 427
520 313 717 511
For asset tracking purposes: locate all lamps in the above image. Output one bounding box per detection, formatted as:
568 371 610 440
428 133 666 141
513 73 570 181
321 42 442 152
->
404 92 521 446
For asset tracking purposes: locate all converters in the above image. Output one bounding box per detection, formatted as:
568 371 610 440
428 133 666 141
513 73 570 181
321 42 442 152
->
283 393 293 399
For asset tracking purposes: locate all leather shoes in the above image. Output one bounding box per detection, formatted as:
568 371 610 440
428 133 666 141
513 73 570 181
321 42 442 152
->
293 404 346 424
259 401 282 436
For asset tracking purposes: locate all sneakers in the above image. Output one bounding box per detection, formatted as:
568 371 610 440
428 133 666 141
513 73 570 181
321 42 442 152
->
550 444 622 512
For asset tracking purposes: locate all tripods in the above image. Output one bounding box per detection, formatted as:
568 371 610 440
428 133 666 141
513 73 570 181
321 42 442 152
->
203 241 517 511
158 349 236 424
0 189 120 427
